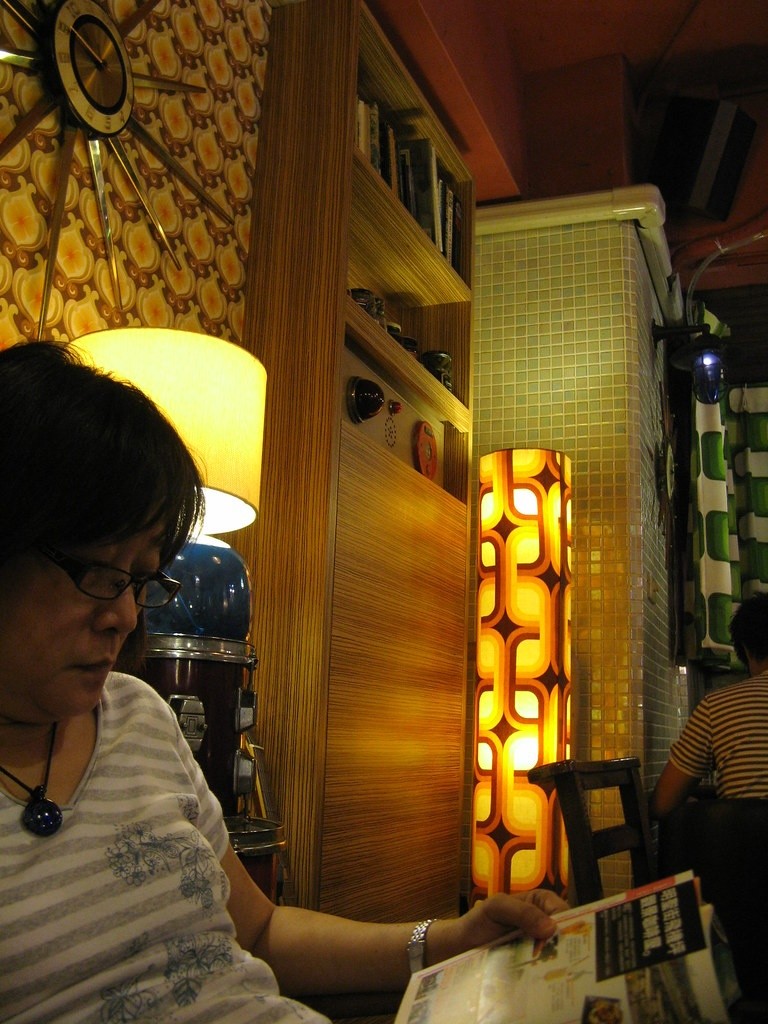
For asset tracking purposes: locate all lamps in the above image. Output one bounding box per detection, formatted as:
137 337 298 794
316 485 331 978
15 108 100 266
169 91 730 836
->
648 318 727 404
63 326 268 821
469 448 577 920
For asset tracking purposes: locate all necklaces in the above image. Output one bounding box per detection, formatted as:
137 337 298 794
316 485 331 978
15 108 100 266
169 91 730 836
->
1 722 62 835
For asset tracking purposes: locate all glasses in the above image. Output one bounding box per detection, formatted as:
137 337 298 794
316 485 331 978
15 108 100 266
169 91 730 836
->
31 535 181 609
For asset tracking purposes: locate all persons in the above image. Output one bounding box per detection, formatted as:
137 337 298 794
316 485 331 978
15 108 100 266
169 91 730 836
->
648 591 768 822
0 337 571 1024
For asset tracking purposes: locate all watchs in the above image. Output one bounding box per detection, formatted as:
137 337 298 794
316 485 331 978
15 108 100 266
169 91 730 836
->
404 918 439 976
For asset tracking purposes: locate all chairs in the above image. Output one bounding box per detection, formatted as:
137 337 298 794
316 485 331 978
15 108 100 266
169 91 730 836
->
650 793 768 1024
531 756 658 912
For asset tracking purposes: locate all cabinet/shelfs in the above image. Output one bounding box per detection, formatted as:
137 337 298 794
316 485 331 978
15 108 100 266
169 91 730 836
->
230 0 476 953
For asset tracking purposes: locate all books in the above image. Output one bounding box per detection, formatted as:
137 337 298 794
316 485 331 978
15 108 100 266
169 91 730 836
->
395 870 738 1024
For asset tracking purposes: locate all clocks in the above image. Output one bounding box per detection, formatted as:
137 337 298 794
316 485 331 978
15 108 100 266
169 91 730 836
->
44 0 134 139
